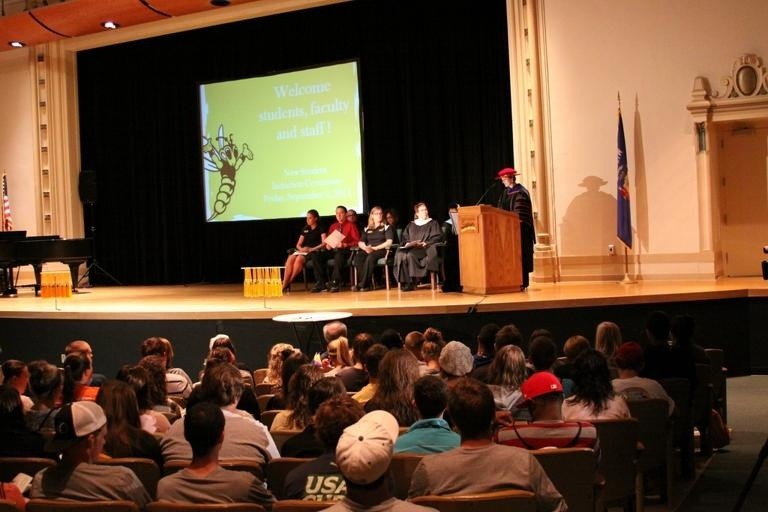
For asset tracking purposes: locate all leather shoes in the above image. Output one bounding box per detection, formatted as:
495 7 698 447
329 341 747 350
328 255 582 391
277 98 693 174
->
351 286 359 291
312 281 325 291
330 279 339 292
361 288 369 291
404 284 413 290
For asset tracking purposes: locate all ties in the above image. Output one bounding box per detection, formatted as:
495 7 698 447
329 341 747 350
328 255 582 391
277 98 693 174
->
338 225 342 234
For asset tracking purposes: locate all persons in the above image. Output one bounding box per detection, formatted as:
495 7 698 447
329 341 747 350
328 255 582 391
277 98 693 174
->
96 379 165 476
315 410 440 512
364 348 421 426
23 360 62 433
346 209 357 222
639 309 698 379
472 323 501 368
116 365 171 436
284 393 397 502
311 321 348 370
322 336 352 378
469 324 523 383
0 359 34 415
492 371 602 467
282 209 326 292
0 384 45 457
421 328 473 375
378 327 421 348
269 364 307 392
202 334 236 362
334 332 377 392
529 329 552 345
487 344 527 409
668 314 711 383
281 378 347 458
138 356 184 423
141 337 192 400
310 206 361 292
405 376 562 512
526 336 554 372
269 364 323 431
351 343 388 403
611 341 675 417
494 167 536 288
156 401 279 512
166 362 281 459
554 335 592 380
393 376 461 454
57 350 101 401
376 208 404 288
392 202 444 291
560 349 631 421
197 362 280 423
268 342 311 363
351 205 394 291
442 208 458 292
30 401 153 510
64 339 106 386
594 322 623 380
408 348 421 372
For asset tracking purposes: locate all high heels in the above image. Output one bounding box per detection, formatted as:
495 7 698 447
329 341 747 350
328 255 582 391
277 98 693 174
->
282 283 291 292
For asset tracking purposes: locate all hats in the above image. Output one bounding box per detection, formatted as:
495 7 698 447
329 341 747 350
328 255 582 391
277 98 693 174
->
618 341 644 361
477 324 500 343
45 401 107 453
516 372 564 408
336 410 400 484
494 168 521 179
438 340 474 376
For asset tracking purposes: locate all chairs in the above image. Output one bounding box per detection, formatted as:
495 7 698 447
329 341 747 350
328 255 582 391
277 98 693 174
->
324 231 362 290
351 229 402 290
286 248 314 290
395 226 446 290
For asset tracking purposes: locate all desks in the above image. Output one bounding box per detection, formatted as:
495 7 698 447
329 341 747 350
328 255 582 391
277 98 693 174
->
271 311 353 354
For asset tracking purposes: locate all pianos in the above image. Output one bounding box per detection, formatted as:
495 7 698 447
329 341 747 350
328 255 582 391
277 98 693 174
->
0 231 95 295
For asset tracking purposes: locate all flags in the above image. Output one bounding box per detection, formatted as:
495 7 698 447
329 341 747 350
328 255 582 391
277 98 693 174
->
616 109 633 248
2 175 13 232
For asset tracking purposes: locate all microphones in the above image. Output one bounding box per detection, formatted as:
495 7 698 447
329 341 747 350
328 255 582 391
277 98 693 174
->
475 180 500 205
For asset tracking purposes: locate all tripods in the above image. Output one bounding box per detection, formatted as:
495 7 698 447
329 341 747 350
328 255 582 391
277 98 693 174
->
75 205 123 287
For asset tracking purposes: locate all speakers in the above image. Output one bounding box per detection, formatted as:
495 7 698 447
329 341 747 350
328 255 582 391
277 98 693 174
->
78 169 96 203
